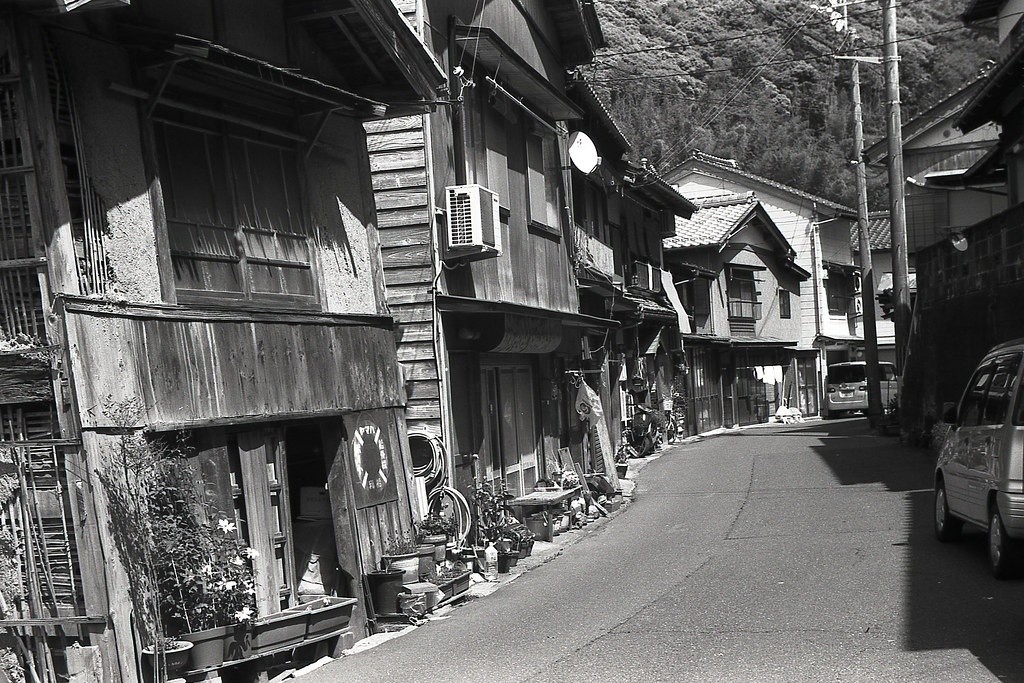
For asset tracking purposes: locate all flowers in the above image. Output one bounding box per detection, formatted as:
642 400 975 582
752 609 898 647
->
163 471 265 633
383 527 426 555
304 598 333 612
414 512 460 536
409 517 428 548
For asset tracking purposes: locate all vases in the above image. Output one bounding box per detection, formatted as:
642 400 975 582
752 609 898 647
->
223 623 252 661
381 551 420 585
179 626 226 670
252 612 311 655
282 596 359 640
411 545 435 572
422 534 446 561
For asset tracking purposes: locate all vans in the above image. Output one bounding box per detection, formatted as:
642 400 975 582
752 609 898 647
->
824 361 898 419
932 338 1024 579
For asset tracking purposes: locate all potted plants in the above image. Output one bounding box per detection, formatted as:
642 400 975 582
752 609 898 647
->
419 563 455 601
367 548 407 615
461 476 535 572
97 392 203 683
437 559 472 595
615 445 628 480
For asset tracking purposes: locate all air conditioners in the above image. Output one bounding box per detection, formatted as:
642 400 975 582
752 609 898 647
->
849 276 861 293
444 184 504 252
848 297 862 315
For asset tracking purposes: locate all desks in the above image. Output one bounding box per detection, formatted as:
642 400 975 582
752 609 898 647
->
509 484 583 542
